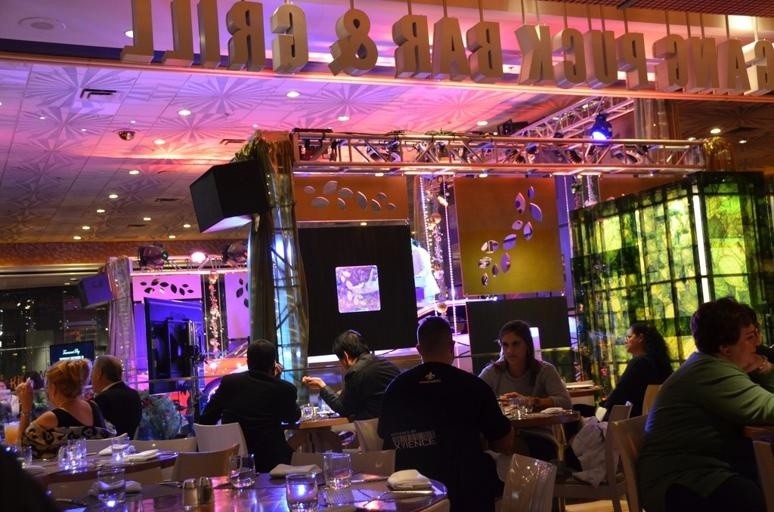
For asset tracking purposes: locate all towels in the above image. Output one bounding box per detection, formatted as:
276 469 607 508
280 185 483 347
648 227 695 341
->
128 448 161 461
386 467 432 489
92 479 142 497
269 463 322 479
541 408 567 413
99 444 127 455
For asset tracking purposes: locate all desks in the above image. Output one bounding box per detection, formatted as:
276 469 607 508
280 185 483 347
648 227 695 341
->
503 408 581 512
745 426 774 511
58 472 449 512
282 415 353 451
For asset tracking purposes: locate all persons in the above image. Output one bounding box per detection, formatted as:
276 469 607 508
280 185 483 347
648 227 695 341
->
23 372 48 411
0 380 19 417
14 359 106 458
601 322 674 423
638 298 774 508
741 322 774 391
479 321 573 511
302 330 402 420
379 317 512 511
196 338 302 471
89 355 142 440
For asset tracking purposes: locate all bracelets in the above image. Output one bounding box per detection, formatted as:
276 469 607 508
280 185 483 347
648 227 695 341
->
19 409 34 417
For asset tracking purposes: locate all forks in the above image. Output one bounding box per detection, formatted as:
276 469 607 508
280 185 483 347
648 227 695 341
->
357 487 433 500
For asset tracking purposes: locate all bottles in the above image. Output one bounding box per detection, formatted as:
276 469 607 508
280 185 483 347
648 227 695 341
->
181 478 198 507
198 476 214 505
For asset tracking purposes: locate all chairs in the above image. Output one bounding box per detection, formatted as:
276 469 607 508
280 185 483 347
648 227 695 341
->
172 443 241 482
192 423 248 457
552 400 633 512
607 414 648 511
500 453 558 512
291 452 350 475
85 433 128 454
642 383 663 415
342 448 396 478
354 417 385 451
129 439 197 454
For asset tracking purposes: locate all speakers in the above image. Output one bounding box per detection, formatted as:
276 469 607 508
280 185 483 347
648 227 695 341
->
190 162 268 232
77 273 113 308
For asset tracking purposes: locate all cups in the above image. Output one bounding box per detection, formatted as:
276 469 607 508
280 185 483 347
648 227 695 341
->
497 397 528 421
111 437 130 462
97 467 126 504
9 445 33 469
286 471 319 512
302 402 314 421
4 423 19 447
59 437 87 470
324 454 352 489
228 453 256 489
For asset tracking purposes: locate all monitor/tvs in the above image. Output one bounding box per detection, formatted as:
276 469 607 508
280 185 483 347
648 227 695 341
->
50 340 95 366
144 296 205 394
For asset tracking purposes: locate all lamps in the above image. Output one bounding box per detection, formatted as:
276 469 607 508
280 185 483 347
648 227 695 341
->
591 114 613 145
